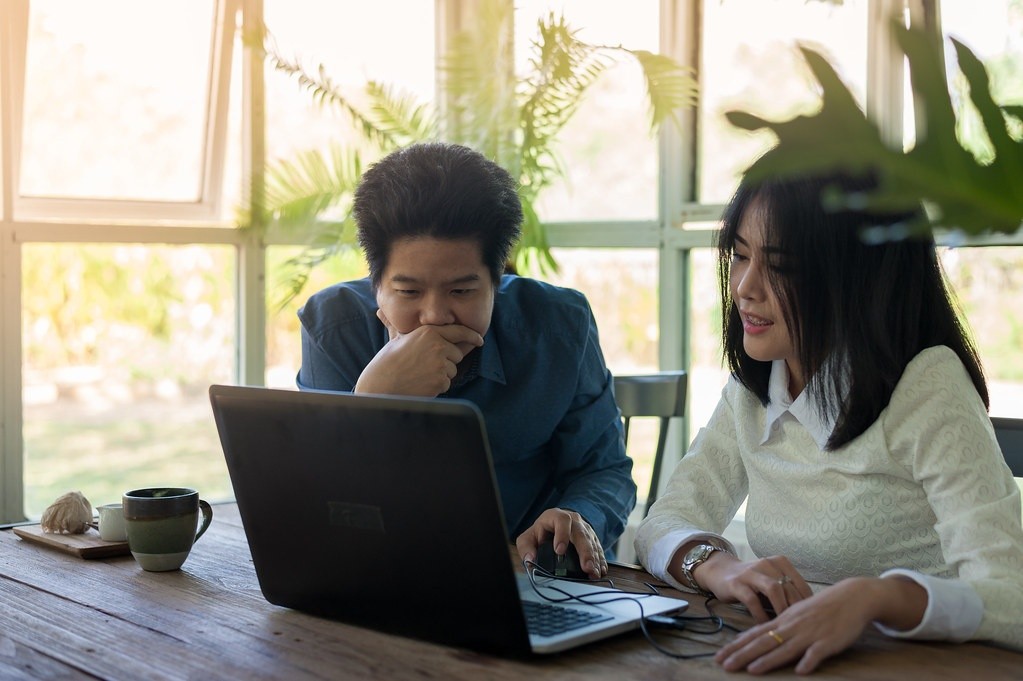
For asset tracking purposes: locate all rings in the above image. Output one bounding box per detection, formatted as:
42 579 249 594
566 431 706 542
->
767 629 784 644
776 574 792 587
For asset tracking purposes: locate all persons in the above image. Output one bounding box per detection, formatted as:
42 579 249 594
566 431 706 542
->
629 146 1023 679
294 144 637 582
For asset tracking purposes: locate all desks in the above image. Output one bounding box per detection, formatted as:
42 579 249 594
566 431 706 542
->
0 489 1019 680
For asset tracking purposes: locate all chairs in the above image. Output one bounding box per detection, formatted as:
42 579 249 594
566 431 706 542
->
608 369 687 575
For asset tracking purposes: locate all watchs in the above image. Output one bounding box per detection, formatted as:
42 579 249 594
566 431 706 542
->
681 543 733 595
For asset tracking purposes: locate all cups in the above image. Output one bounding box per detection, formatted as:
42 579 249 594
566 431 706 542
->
123 487 213 571
97 502 125 541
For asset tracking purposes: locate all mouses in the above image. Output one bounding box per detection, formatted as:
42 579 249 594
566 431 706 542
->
537 536 588 580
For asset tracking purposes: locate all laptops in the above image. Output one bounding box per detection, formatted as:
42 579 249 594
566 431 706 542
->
206 385 689 662
